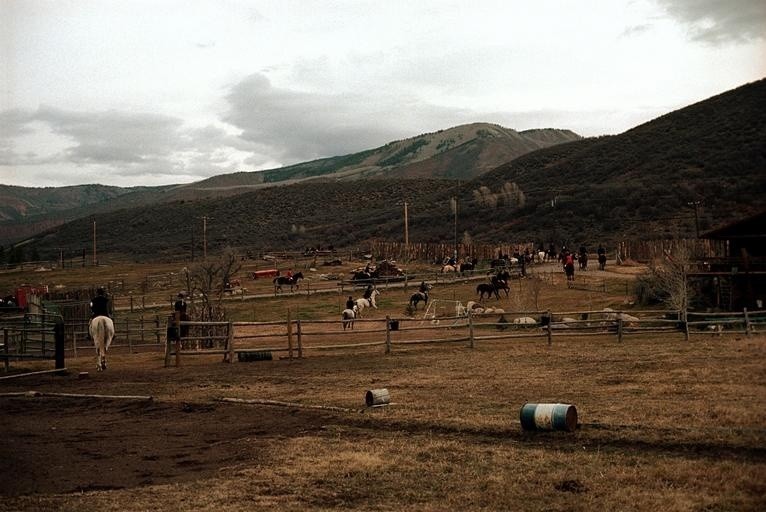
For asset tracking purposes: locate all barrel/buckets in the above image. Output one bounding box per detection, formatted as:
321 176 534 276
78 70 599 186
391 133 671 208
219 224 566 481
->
519 401 579 433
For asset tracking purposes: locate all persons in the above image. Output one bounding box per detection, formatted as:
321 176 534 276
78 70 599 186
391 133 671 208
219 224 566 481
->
173 292 187 320
419 281 429 299
287 268 294 281
86 287 116 341
363 284 372 307
344 296 358 318
448 240 612 284
365 263 373 278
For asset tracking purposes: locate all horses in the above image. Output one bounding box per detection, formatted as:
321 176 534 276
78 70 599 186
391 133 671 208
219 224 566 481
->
492 270 510 296
88 315 115 372
272 272 304 294
563 258 576 281
477 284 502 301
599 255 606 271
355 288 380 319
441 265 456 277
578 252 588 272
166 314 189 349
342 304 358 329
410 283 432 311
